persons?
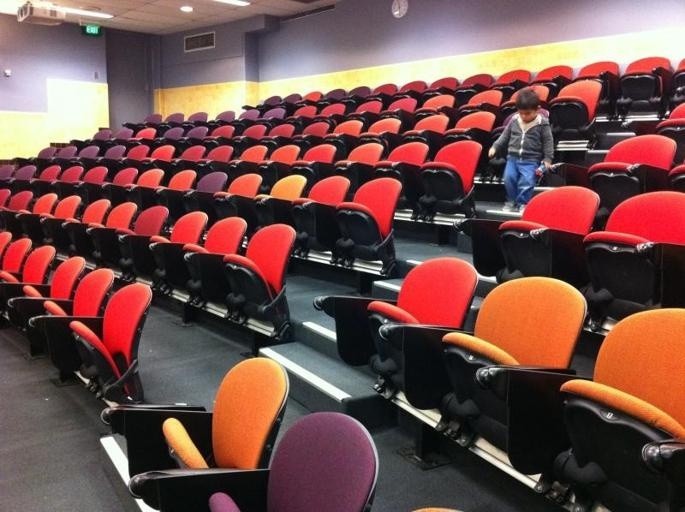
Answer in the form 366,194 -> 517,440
486,89 -> 553,214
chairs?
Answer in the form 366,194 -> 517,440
0,55 -> 685,512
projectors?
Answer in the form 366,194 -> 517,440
17,1 -> 66,26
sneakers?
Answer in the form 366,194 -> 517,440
503,203 -> 519,212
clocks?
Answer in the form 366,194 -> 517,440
389,0 -> 410,19
